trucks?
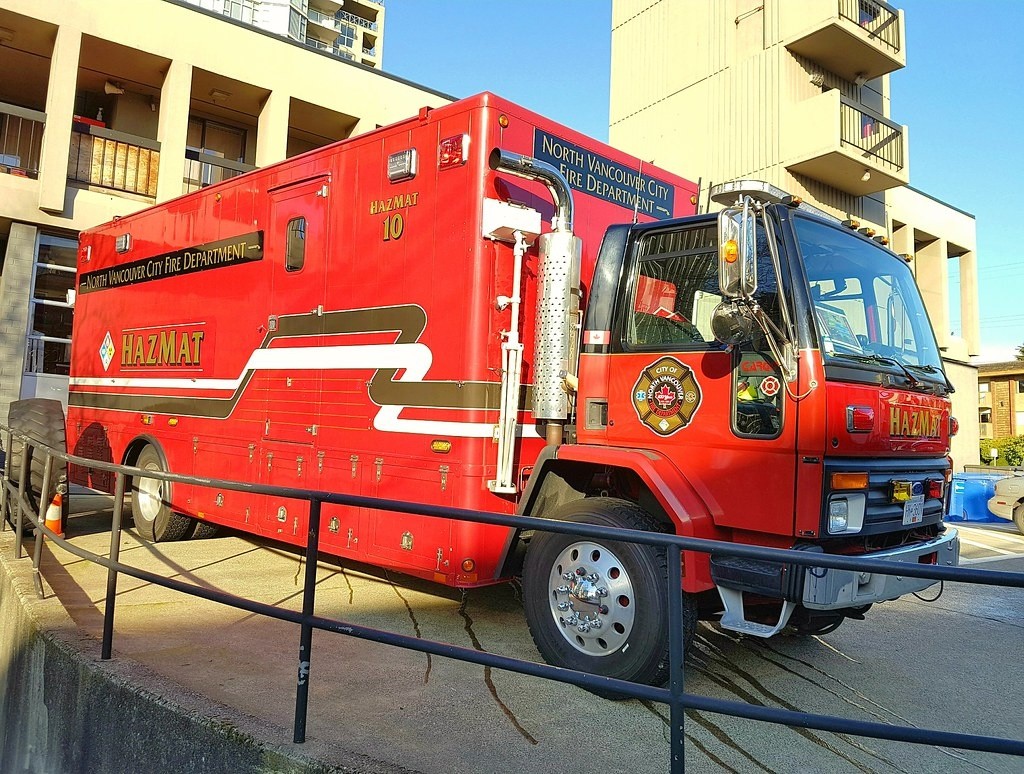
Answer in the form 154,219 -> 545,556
59,88 -> 965,703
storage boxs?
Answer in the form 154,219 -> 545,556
0,154 -> 20,168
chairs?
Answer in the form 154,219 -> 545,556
635,276 -> 705,344
